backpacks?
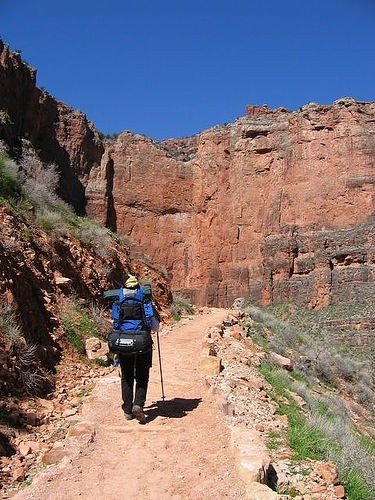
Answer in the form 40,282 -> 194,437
108,285 -> 153,353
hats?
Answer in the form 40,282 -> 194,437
125,276 -> 138,287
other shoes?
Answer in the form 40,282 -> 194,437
124,412 -> 133,420
131,405 -> 147,425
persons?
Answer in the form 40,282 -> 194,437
112,276 -> 159,424
